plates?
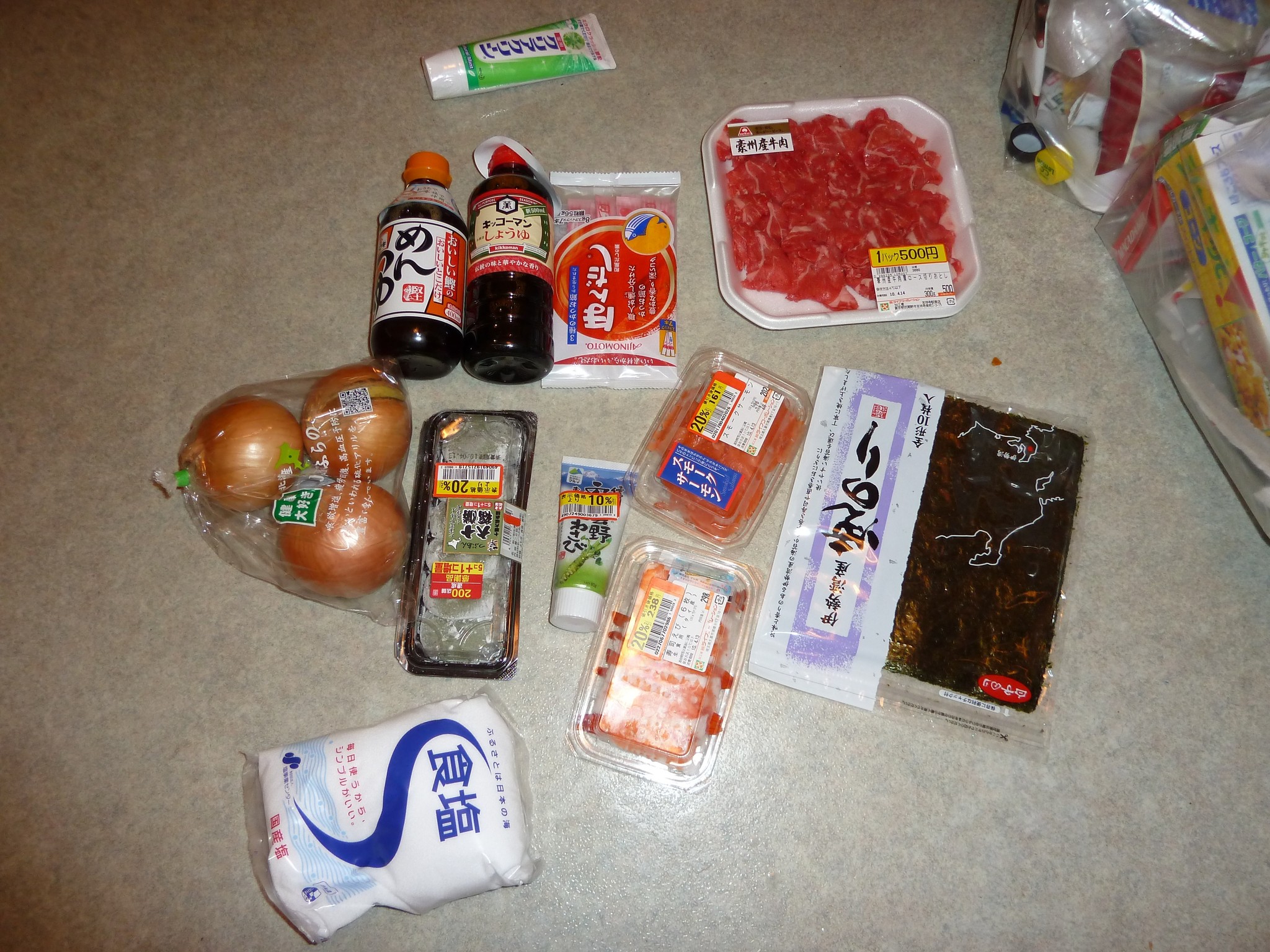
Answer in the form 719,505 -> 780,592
701,95 -> 983,331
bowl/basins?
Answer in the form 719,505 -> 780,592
622,343 -> 814,560
562,534 -> 765,792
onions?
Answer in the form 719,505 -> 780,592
177,396 -> 303,512
275,479 -> 410,596
299,364 -> 412,484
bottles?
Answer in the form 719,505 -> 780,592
461,143 -> 555,386
368,150 -> 467,380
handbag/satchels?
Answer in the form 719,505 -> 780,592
998,1 -> 1270,218
1093,86 -> 1270,546
